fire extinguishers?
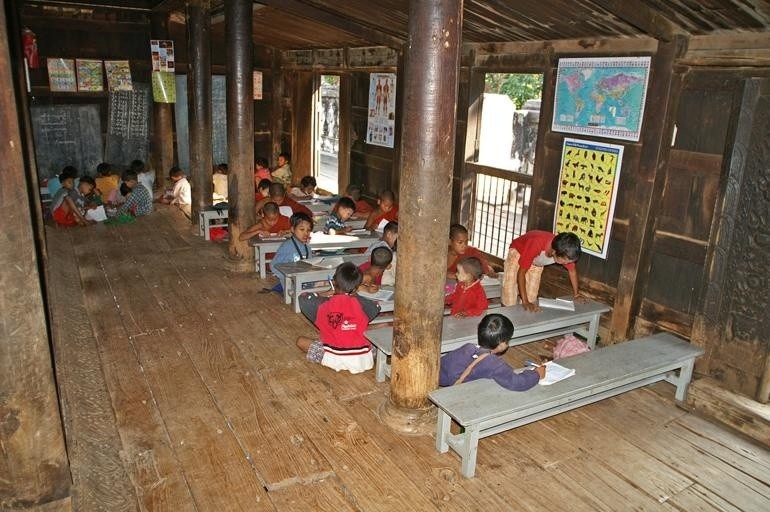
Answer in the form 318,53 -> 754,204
21,24 -> 40,69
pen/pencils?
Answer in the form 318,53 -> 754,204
526,360 -> 541,368
329,280 -> 335,290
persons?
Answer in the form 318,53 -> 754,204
357,221 -> 495,319
500,230 -> 591,313
162,166 -> 191,205
322,184 -> 374,234
363,191 -> 399,231
239,154 -> 317,272
269,211 -> 331,297
213,163 -> 228,199
47,158 -> 155,229
297,262 -> 382,375
440,313 -> 547,392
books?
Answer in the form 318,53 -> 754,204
525,359 -> 577,387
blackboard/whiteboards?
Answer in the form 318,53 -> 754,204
175,75 -> 228,175
104,82 -> 152,167
30,104 -> 103,183
